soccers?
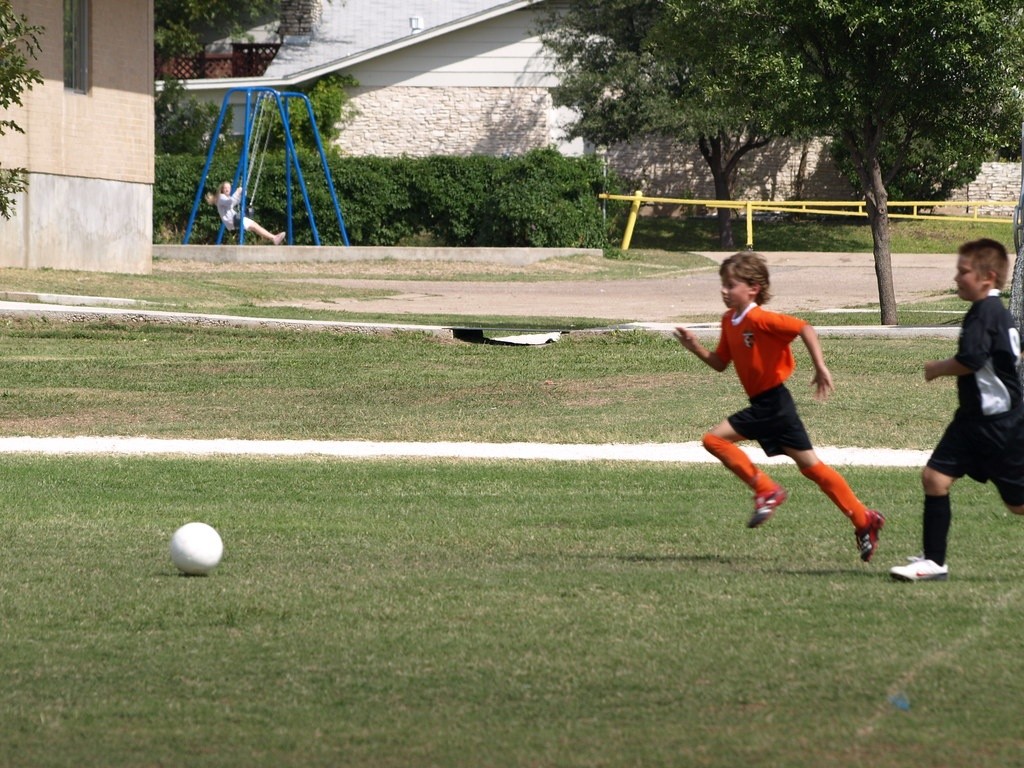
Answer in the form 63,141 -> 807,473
167,521 -> 224,575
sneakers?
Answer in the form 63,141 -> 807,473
889,552 -> 948,581
854,510 -> 885,562
746,485 -> 785,528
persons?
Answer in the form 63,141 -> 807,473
671,251 -> 885,562
888,237 -> 1024,583
213,181 -> 286,245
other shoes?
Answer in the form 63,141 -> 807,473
274,232 -> 286,245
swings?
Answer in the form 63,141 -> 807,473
228,96 -> 277,233
